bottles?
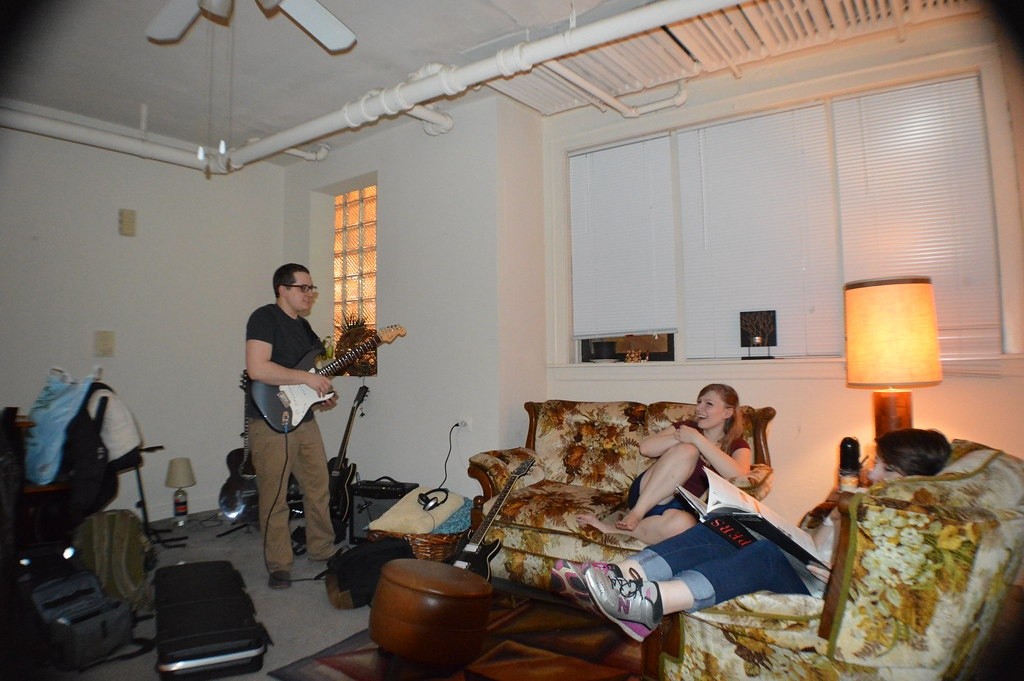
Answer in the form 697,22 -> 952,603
837,469 -> 858,493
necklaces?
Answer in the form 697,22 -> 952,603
700,455 -> 710,466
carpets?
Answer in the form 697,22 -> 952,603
265,572 -> 682,680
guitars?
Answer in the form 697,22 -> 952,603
442,460 -> 534,579
219,369 -> 260,525
250,324 -> 407,433
328,386 -> 368,519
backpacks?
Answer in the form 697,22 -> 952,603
314,534 -> 417,610
18,548 -> 154,675
74,510 -> 151,604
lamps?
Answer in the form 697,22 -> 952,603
164,455 -> 198,522
840,274 -> 940,437
199,0 -> 286,20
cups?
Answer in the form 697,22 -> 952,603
592,341 -> 617,360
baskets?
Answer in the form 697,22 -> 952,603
363,528 -> 470,562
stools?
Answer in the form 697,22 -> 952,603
365,557 -> 495,667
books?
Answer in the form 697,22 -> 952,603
675,466 -> 833,582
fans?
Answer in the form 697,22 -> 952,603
143,0 -> 360,55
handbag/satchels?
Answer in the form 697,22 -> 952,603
23,366 -> 95,488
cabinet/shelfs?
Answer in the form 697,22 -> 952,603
15,412 -> 62,494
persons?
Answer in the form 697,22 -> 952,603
245,263 -> 340,588
551,428 -> 953,641
574,383 -> 751,545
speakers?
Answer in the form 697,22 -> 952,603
349,475 -> 419,546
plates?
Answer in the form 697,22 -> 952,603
589,359 -> 620,362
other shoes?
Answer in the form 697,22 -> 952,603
267,569 -> 292,590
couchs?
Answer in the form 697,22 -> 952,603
659,436 -> 1024,681
468,398 -> 777,609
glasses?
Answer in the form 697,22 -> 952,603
281,284 -> 318,293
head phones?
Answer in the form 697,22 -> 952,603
418,488 -> 448,511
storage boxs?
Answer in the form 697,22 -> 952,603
23,567 -> 135,668
152,560 -> 276,681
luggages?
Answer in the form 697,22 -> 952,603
155,561 -> 276,681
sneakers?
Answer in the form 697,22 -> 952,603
551,559 -> 623,616
585,567 -> 664,643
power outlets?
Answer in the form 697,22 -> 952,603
92,331 -> 117,359
119,208 -> 138,236
456,416 -> 473,432
